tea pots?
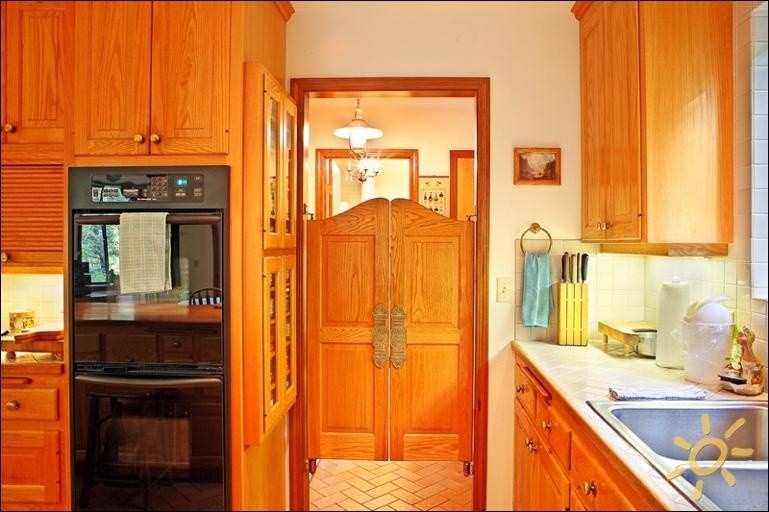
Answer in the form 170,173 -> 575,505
683,295 -> 736,386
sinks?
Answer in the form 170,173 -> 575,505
651,461 -> 769,512
586,397 -> 768,461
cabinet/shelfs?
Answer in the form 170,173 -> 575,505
0,1 -> 295,511
511,2 -> 769,512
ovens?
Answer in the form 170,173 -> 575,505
68,166 -> 229,512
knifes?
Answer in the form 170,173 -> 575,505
561,251 -> 589,283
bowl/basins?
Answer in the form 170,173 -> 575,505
632,331 -> 657,357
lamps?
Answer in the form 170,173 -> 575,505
333,97 -> 383,185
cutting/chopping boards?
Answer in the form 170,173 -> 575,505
14,321 -> 64,344
